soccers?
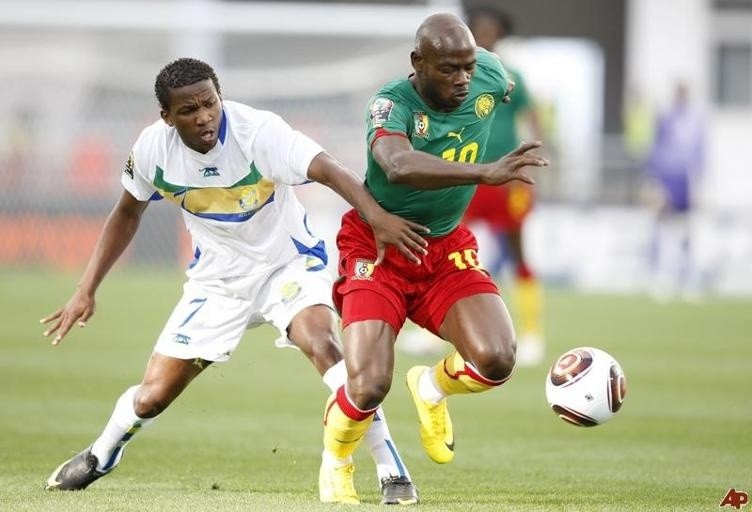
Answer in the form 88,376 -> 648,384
545,346 -> 626,427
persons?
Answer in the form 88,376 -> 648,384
633,71 -> 710,298
39,57 -> 432,506
392,6 -> 551,369
314,12 -> 551,507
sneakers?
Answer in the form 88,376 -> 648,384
380,475 -> 419,506
405,365 -> 454,463
45,444 -> 124,491
318,458 -> 358,503
